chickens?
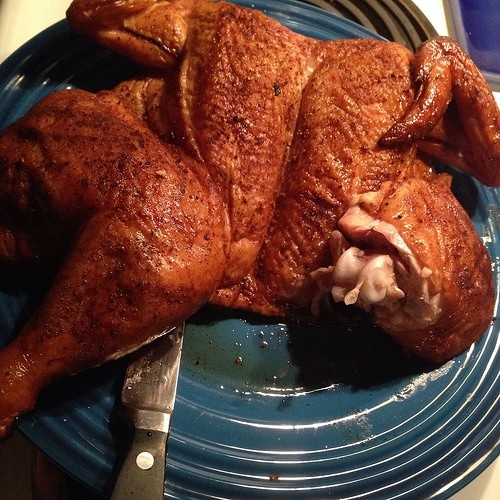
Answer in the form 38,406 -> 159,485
0,0 -> 500,436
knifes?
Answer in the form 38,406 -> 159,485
110,321 -> 185,500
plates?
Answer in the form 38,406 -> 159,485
1,1 -> 499,499
